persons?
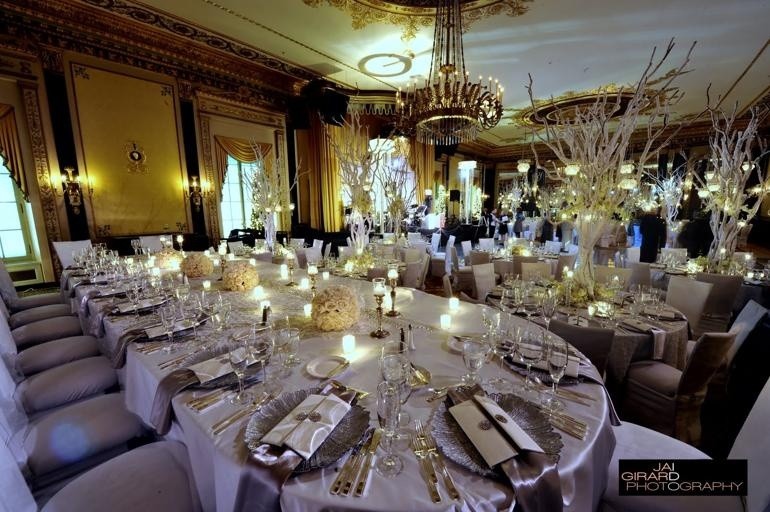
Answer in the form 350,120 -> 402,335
414,212 -> 421,226
445,217 -> 449,228
540,200 -> 579,243
449,215 -> 459,228
480,206 -> 525,238
679,209 -> 715,257
638,205 -> 668,263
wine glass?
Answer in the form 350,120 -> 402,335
67,234 -> 597,506
652,252 -> 769,285
599,270 -> 676,337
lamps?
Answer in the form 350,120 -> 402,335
51,167 -> 94,215
393,1 -> 504,147
697,163 -> 756,199
517,126 -> 531,174
182,175 -> 211,213
620,148 -> 634,175
617,174 -> 637,190
